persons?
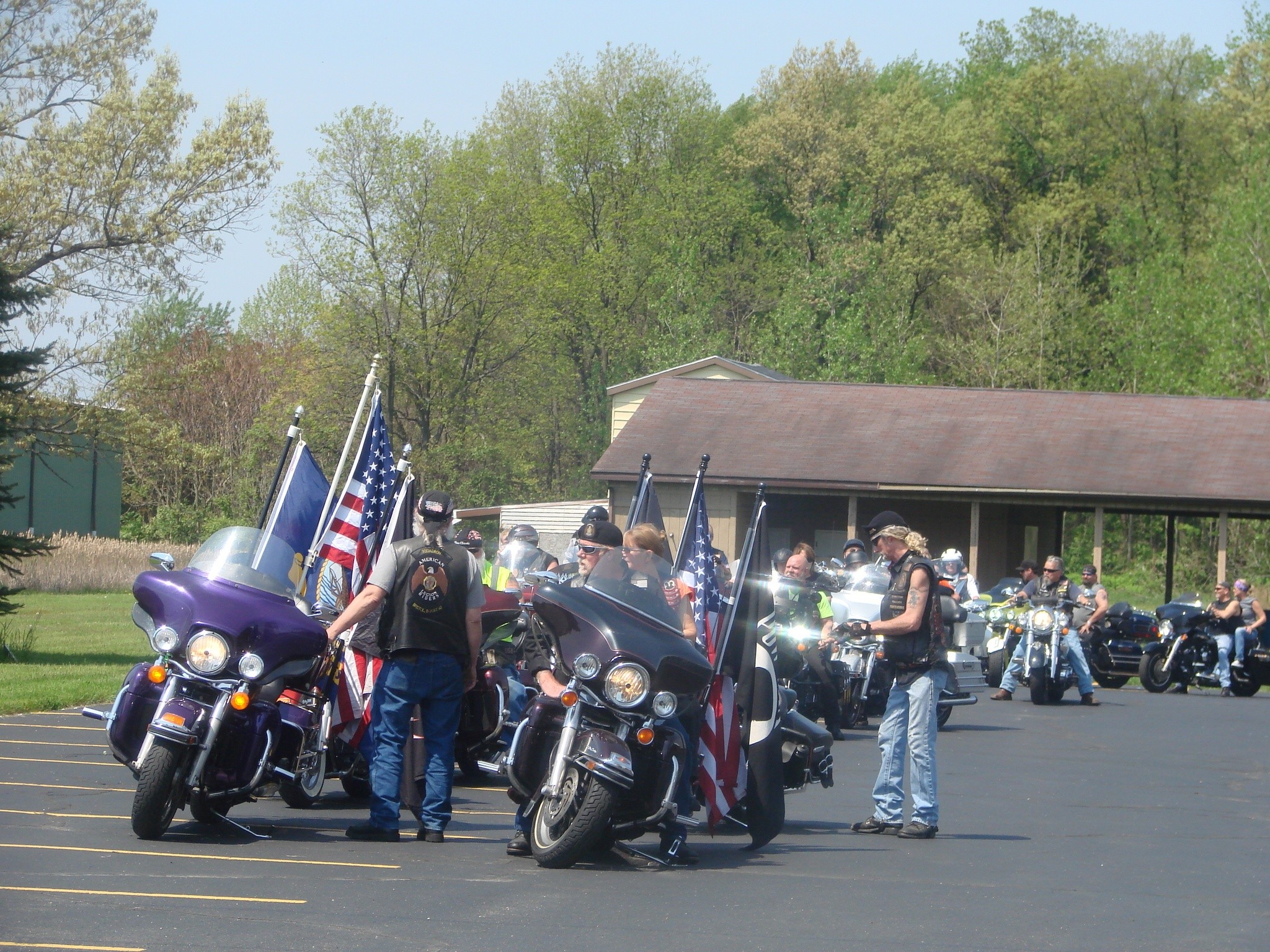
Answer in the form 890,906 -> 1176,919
990,556 -> 1100,706
826,512 -> 948,839
1166,581 -> 1243,697
1231,578 -> 1266,669
708,525 -> 732,584
1016,558 -> 1039,585
772,539 -> 982,741
326,491 -> 485,844
453,505 -> 701,865
1071,565 -> 1108,688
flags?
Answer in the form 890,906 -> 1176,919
252,381 -> 425,822
628,470 -> 786,850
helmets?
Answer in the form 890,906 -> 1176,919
581,506 -> 609,524
940,548 -> 963,567
508,524 -> 538,547
708,525 -> 714,541
845,551 -> 868,566
843,539 -> 865,553
773,548 -> 793,571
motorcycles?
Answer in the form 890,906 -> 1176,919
832,564 -> 988,730
478,546 -> 749,869
80,526 -> 362,841
959,592 -> 1270,706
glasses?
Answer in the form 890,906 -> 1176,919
850,549 -> 861,553
468,548 -> 481,553
871,534 -> 882,546
624,548 -> 643,554
807,558 -> 813,562
1214,588 -> 1225,590
1081,573 -> 1093,576
1043,568 -> 1059,573
578,544 -> 607,555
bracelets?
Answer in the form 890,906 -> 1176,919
1087,621 -> 1092,626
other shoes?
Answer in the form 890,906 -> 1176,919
989,687 -> 1013,701
1231,660 -> 1244,668
1168,686 -> 1188,694
345,821 -> 445,842
851,817 -> 937,839
1081,693 -> 1100,705
690,795 -> 701,812
1221,687 -> 1230,697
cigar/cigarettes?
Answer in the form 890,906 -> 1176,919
874,555 -> 883,566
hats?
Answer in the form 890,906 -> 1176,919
1015,559 -> 1038,571
453,528 -> 483,548
864,511 -> 908,541
417,490 -> 454,521
580,521 -> 623,547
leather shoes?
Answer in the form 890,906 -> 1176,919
660,839 -> 699,863
506,829 -> 532,855
